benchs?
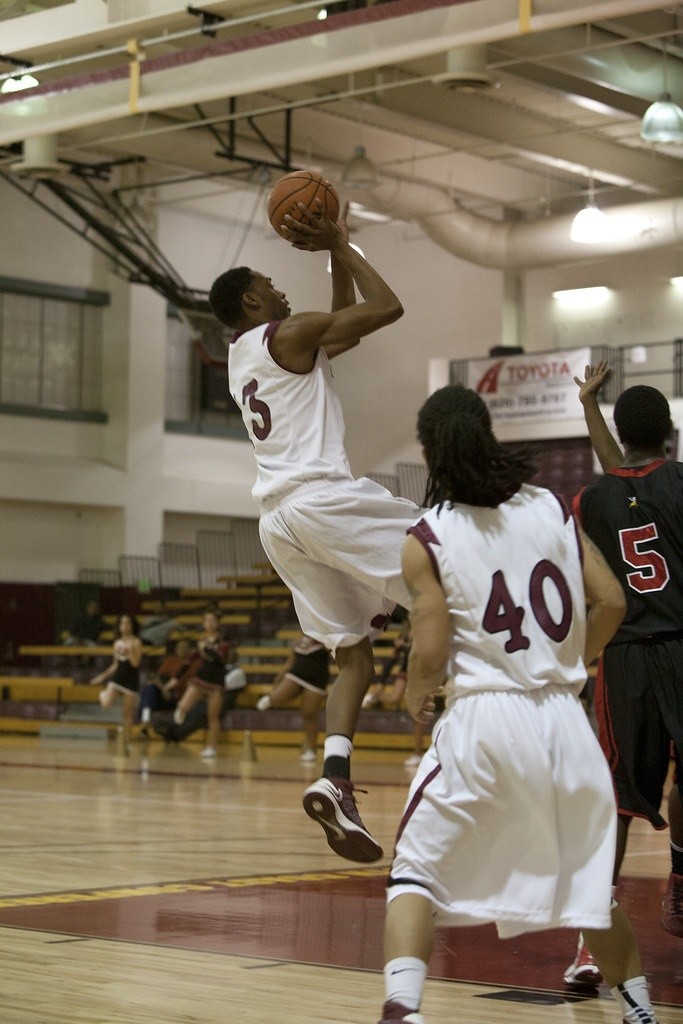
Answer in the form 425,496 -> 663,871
0,561 -> 602,752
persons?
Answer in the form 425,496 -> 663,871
380,618 -> 428,767
572,385 -> 682,939
571,359 -> 624,474
86,609 -> 330,765
205,197 -> 431,867
558,386 -> 683,990
378,385 -> 661,1024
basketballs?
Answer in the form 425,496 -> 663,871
267,170 -> 340,243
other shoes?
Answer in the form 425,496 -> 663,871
377,1002 -> 424,1024
562,943 -> 603,985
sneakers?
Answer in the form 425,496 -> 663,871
660,872 -> 683,938
302,776 -> 383,863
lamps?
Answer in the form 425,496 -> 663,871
640,38 -> 683,143
571,170 -> 606,244
